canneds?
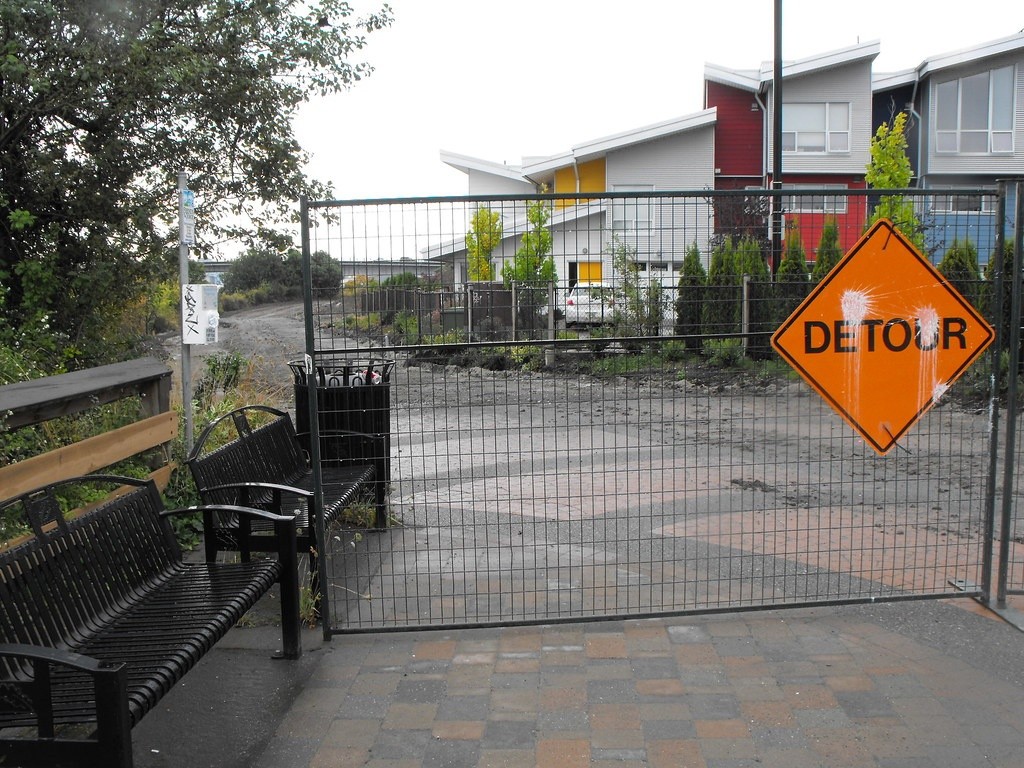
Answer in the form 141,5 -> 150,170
362,369 -> 380,384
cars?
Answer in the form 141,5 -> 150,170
564,281 -> 622,329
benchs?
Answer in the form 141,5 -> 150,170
1,475 -> 303,768
183,405 -> 389,618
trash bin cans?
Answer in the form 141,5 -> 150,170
286,357 -> 397,531
